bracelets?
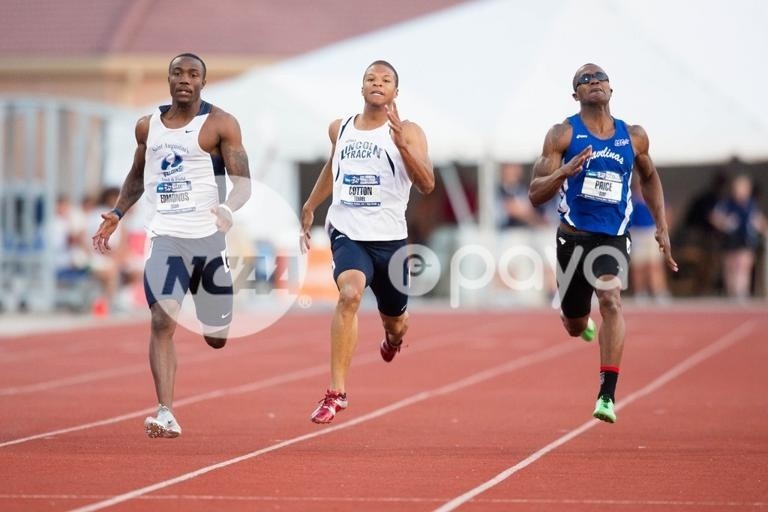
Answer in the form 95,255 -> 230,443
217,201 -> 235,217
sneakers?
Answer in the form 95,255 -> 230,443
592,395 -> 616,423
380,331 -> 403,363
143,411 -> 182,439
311,389 -> 347,424
580,317 -> 596,341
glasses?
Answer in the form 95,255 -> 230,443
575,72 -> 609,92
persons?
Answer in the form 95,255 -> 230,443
528,62 -> 681,424
299,59 -> 438,425
90,53 -> 253,439
53,183 -> 146,314
502,160 -> 767,297
